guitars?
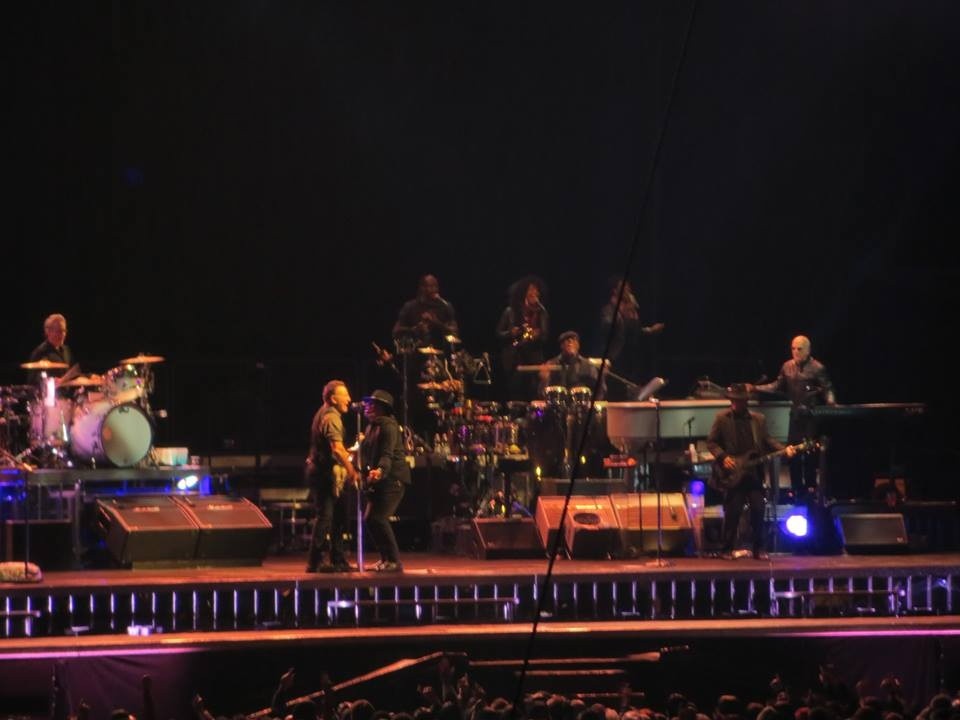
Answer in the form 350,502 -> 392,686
708,435 -> 828,498
644,318 -> 667,335
332,429 -> 365,499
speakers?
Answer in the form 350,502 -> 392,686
472,490 -> 692,560
835,513 -> 909,554
91,494 -> 273,566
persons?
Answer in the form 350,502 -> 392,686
745,333 -> 835,498
0,660 -> 960,720
537,330 -> 608,402
707,382 -> 797,561
25,314 -> 85,401
391,273 -> 459,445
303,380 -> 363,573
598,272 -> 664,402
494,274 -> 549,403
356,389 -> 411,572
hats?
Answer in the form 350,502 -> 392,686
722,382 -> 751,400
363,389 -> 394,412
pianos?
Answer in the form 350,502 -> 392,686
606,400 -> 795,499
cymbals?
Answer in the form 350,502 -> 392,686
446,336 -> 460,342
60,376 -> 103,387
23,359 -> 67,370
119,356 -> 166,365
413,345 -> 436,356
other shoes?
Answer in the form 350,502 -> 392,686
373,561 -> 403,572
720,551 -> 736,559
91,559 -> 131,570
754,551 -> 770,561
306,564 -> 335,573
334,564 -> 353,572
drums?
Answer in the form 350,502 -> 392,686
100,403 -> 152,468
113,376 -> 147,401
29,398 -> 71,448
542,385 -> 568,412
71,400 -> 118,464
570,385 -> 592,414
461,415 -> 493,465
495,418 -> 531,463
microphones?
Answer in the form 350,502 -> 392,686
683,417 -> 695,425
432,293 -> 447,306
351,401 -> 366,410
